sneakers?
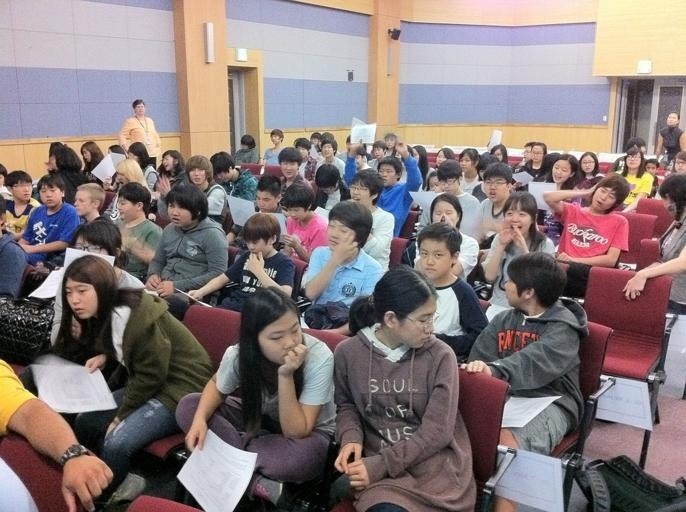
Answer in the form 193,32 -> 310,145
106,472 -> 146,504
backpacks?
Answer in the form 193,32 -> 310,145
576,455 -> 686,512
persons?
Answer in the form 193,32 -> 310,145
1,128 -> 686,510
654,112 -> 685,160
116,99 -> 162,169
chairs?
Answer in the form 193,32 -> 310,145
0,149 -> 686,512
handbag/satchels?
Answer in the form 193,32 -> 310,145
0,295 -> 54,365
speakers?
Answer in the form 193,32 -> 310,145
391,28 -> 401,40
386,46 -> 393,75
206,22 -> 214,63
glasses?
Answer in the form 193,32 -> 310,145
501,280 -> 509,288
70,242 -> 102,253
627,155 -> 642,161
484,179 -> 505,187
439,179 -> 456,185
674,161 -> 686,166
317,186 -> 333,194
405,312 -> 440,329
349,184 -> 368,193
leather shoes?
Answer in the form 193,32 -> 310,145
276,479 -> 331,512
171,449 -> 192,468
